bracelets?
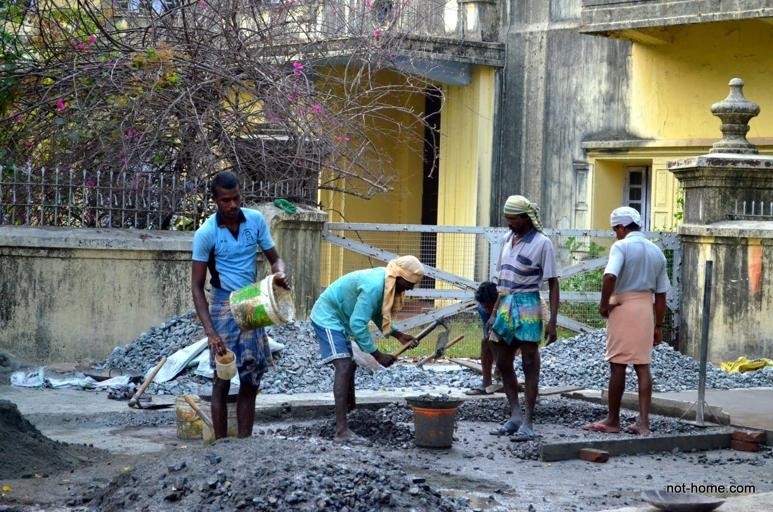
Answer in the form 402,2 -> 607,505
655,323 -> 662,328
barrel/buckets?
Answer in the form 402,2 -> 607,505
200,400 -> 239,440
414,406 -> 456,450
174,396 -> 202,439
229,274 -> 295,334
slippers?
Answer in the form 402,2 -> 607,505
489,421 -> 545,442
465,387 -> 494,395
581,418 -> 651,436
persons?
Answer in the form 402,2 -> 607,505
462,280 -> 523,395
579,205 -> 671,438
306,253 -> 426,448
188,170 -> 293,441
485,193 -> 561,443
